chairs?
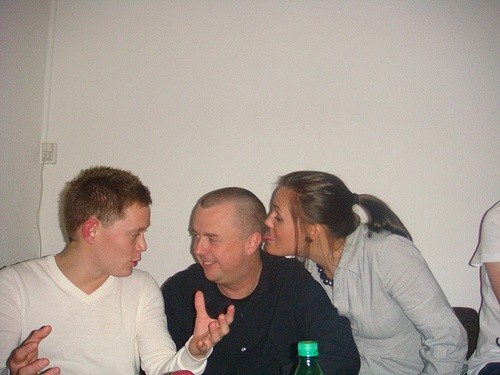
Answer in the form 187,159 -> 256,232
452,307 -> 479,362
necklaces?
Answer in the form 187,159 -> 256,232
316,264 -> 333,286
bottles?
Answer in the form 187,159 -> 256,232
294,341 -> 323,375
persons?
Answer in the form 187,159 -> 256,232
0,167 -> 235,375
140,187 -> 361,375
469,201 -> 500,375
262,171 -> 468,375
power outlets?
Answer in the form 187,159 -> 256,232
40,143 -> 57,164
